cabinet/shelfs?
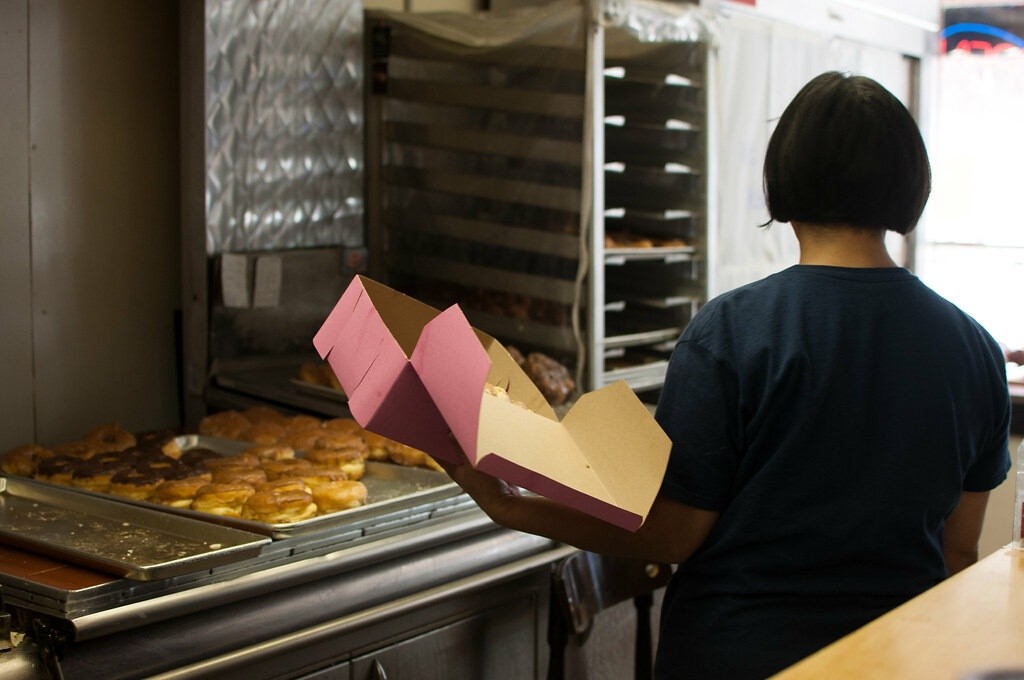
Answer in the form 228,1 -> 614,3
298,593 -> 539,680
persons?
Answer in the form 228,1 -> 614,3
429,72 -> 1013,680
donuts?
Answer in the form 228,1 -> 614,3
0,405 -> 445,528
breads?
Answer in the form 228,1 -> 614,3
605,229 -> 691,250
297,345 -> 574,406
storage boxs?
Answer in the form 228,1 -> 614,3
311,272 -> 672,533
